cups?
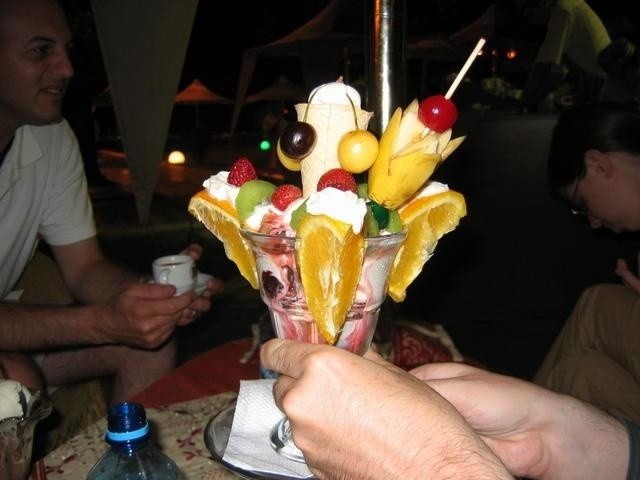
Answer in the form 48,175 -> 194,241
149,254 -> 198,299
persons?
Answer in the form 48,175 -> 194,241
2,0 -> 227,459
259,338 -> 640,479
528,89 -> 640,427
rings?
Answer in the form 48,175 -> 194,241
191,308 -> 196,319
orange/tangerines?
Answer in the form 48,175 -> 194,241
386,187 -> 468,304
187,187 -> 263,289
295,209 -> 368,347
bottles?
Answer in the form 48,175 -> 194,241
85,398 -> 185,479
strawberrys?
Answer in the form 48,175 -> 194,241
228,158 -> 257,186
316,168 -> 357,194
272,185 -> 302,211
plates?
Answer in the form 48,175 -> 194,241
144,272 -> 215,299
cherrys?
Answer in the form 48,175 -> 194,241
419,95 -> 456,133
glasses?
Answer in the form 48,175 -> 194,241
566,171 -> 587,221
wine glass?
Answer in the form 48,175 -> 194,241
237,222 -> 409,464
